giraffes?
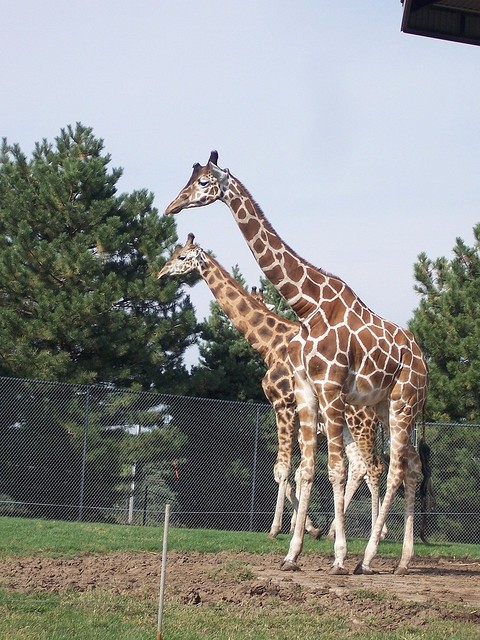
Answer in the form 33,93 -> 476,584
166,150 -> 437,575
156,232 -> 389,543
